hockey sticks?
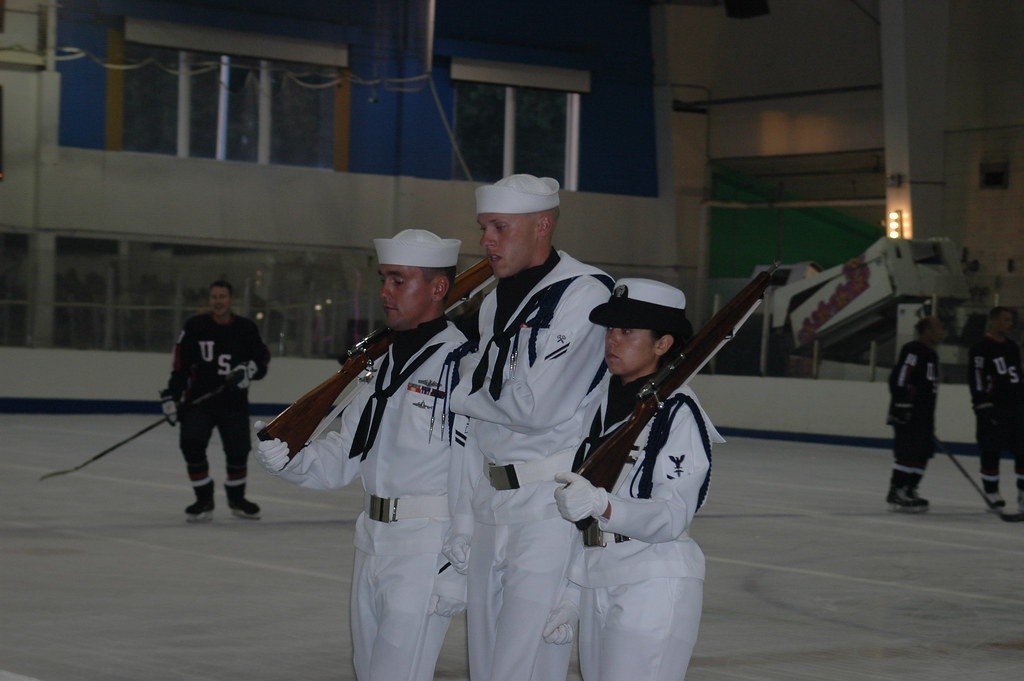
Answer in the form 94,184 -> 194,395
932,434 -> 1023,523
39,383 -> 227,481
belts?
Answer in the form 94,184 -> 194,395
580,517 -> 631,547
362,490 -> 451,523
483,450 -> 577,490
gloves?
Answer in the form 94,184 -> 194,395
229,359 -> 259,391
441,526 -> 474,575
889,397 -> 913,424
973,403 -> 995,428
255,419 -> 290,473
553,471 -> 609,523
424,571 -> 470,618
158,387 -> 181,427
542,598 -> 581,646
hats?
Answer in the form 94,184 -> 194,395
588,279 -> 687,329
376,228 -> 462,267
476,174 -> 560,213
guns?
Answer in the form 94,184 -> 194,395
565,267 -> 775,529
257,258 -> 494,458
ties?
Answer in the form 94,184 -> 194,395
468,284 -> 555,402
349,341 -> 449,462
571,405 -> 641,476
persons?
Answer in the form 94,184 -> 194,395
884,315 -> 945,508
442,173 -> 615,681
254,224 -> 470,680
159,280 -> 271,514
966,307 -> 1024,508
543,277 -> 727,681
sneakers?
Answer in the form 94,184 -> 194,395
1016,489 -> 1023,511
183,480 -> 216,523
223,476 -> 262,519
986,490 -> 1006,513
886,484 -> 932,515
